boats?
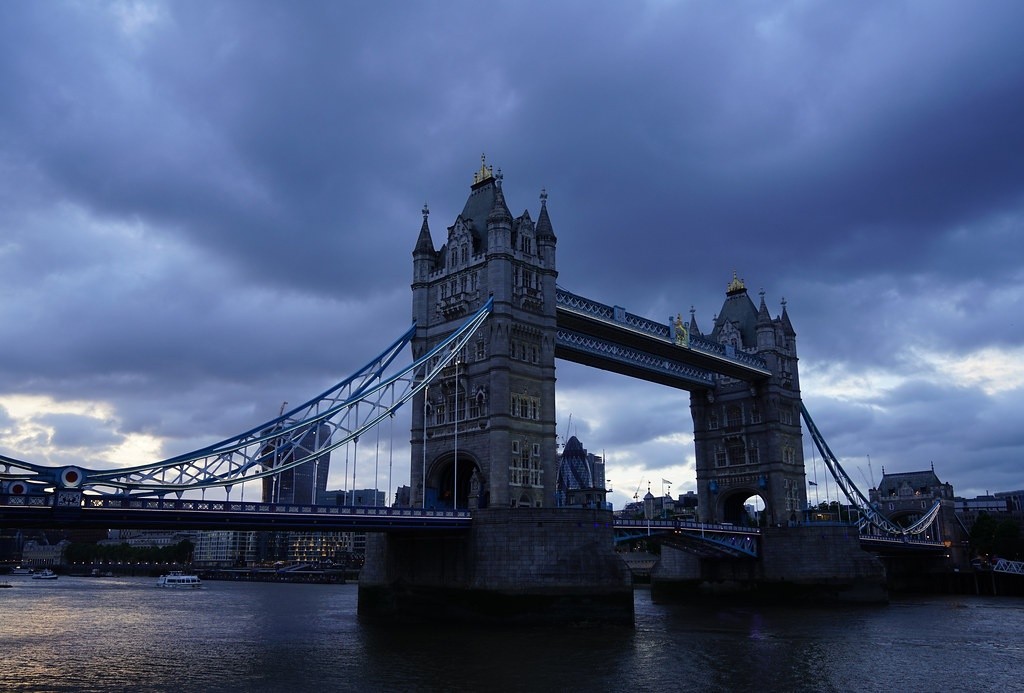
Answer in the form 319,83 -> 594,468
31,568 -> 58,579
156,570 -> 203,591
